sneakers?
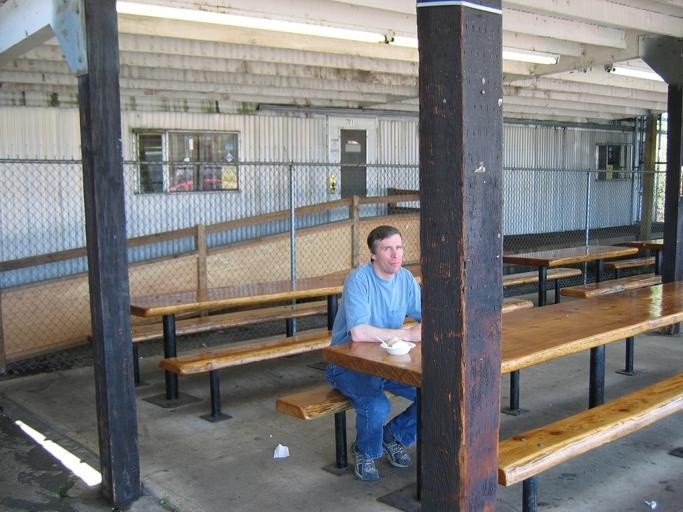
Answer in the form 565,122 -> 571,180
351,441 -> 379,481
382,440 -> 411,468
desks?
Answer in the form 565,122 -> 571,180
130,238 -> 683,512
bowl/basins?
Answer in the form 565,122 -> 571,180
380,341 -> 416,355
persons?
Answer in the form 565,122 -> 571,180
325,225 -> 422,481
